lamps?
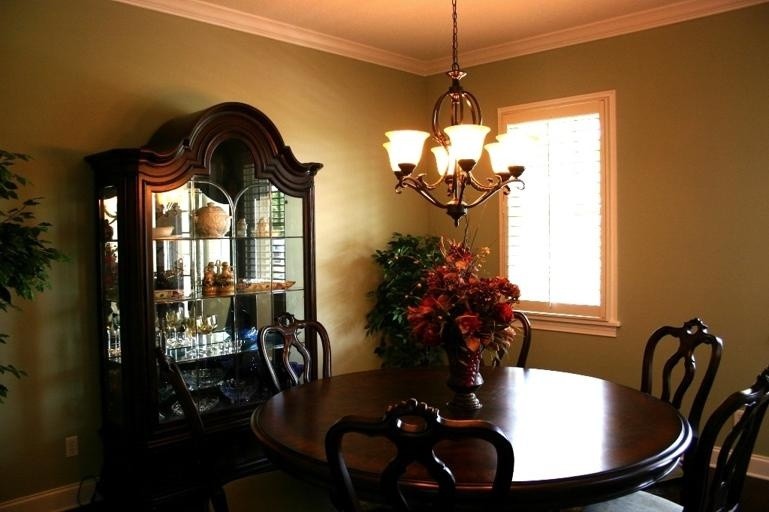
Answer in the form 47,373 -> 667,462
380,0 -> 525,226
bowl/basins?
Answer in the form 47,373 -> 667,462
156,226 -> 174,237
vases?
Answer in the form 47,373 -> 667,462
443,344 -> 485,411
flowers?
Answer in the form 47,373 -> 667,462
405,232 -> 520,357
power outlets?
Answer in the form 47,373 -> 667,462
65,436 -> 79,458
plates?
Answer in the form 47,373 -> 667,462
171,366 -> 258,416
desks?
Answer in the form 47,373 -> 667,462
248,362 -> 696,512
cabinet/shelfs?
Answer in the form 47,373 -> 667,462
82,97 -> 323,484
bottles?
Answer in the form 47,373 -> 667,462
236,218 -> 247,236
171,203 -> 181,235
104,218 -> 113,239
201,262 -> 217,297
217,262 -> 236,297
195,203 -> 232,239
256,218 -> 267,235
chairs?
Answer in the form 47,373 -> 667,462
572,362 -> 769,511
320,396 -> 514,512
489,310 -> 535,367
154,342 -> 337,511
618,315 -> 727,494
255,310 -> 335,394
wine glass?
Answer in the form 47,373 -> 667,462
165,308 -> 219,356
